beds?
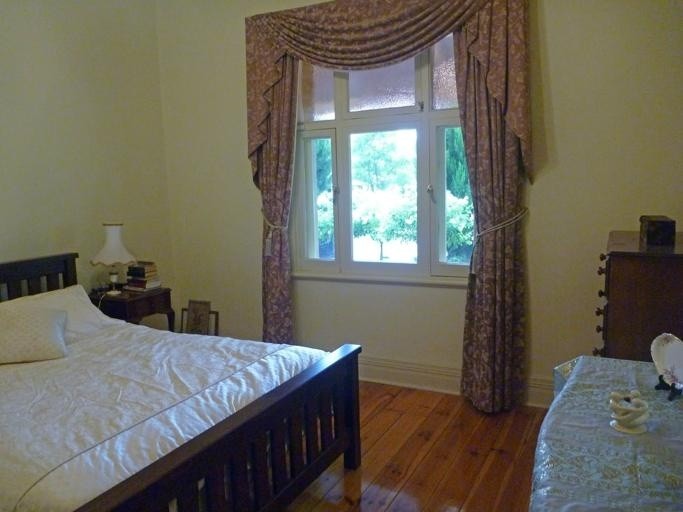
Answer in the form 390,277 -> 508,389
0,253 -> 363,512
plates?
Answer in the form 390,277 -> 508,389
650,332 -> 683,391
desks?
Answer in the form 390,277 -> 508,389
527,353 -> 683,512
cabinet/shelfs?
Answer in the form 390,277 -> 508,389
595,231 -> 683,362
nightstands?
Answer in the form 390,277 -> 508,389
89,287 -> 175,330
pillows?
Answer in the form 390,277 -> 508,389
0,282 -> 123,364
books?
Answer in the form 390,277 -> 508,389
123,261 -> 161,292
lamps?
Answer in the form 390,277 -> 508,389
89,224 -> 137,296
